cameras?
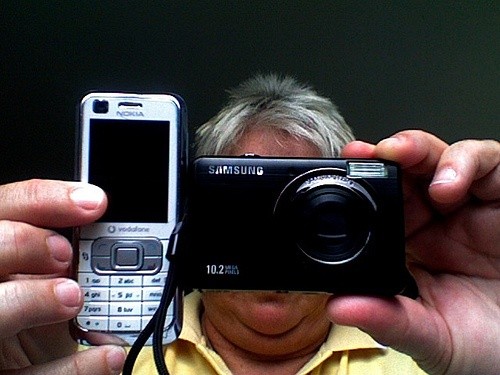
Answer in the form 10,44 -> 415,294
187,157 -> 406,296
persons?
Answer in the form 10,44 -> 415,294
0,69 -> 500,375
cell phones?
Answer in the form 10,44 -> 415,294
70,90 -> 189,349
